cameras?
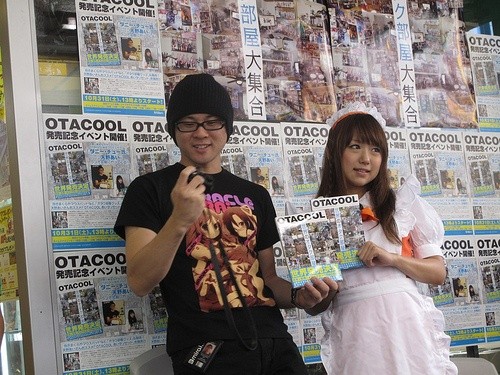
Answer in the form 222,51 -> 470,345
187,171 -> 213,194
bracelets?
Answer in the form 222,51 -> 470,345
290,288 -> 304,309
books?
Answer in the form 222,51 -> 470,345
275,194 -> 366,289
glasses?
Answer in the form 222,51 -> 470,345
174,118 -> 225,132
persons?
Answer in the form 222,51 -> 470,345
128,309 -> 142,330
144,49 -> 158,68
315,102 -> 459,375
116,175 -> 128,195
107,302 -> 120,323
124,38 -> 138,60
454,278 -> 464,297
94,165 -> 109,187
469,285 -> 480,301
114,73 -> 339,375
272,176 -> 284,191
254,169 -> 267,184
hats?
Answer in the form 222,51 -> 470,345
167,74 -> 233,136
